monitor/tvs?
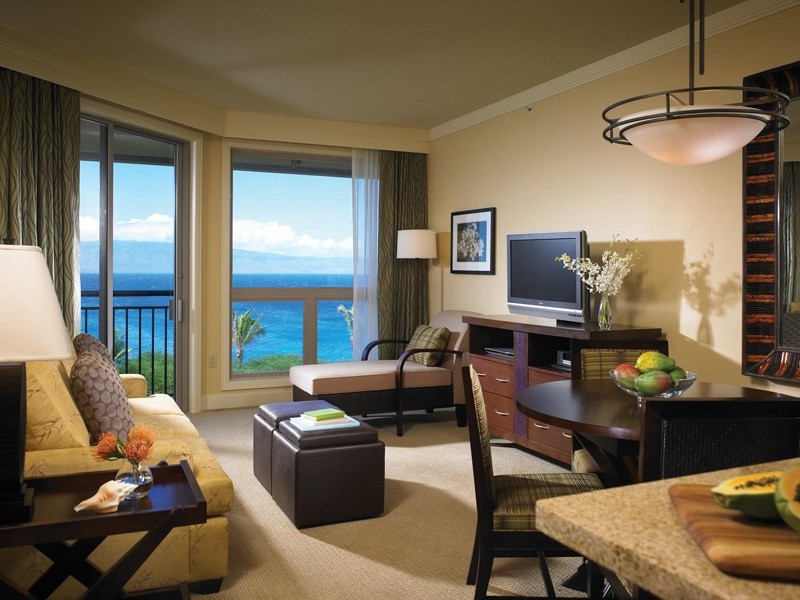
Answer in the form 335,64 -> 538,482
506,230 -> 592,324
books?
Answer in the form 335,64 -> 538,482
289,408 -> 360,433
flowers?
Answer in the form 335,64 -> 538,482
554,234 -> 643,324
89,427 -> 155,484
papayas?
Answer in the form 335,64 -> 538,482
613,352 -> 687,395
709,466 -> 800,535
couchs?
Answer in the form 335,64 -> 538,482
0,332 -> 234,595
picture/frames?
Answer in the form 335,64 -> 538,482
450,207 -> 496,275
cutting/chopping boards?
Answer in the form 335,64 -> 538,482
669,485 -> 800,575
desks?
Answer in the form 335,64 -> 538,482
535,457 -> 800,600
516,378 -> 800,593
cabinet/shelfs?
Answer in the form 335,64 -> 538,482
462,314 -> 662,473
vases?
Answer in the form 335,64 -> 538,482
114,460 -> 154,499
597,293 -> 612,329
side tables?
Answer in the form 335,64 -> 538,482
0,460 -> 206,600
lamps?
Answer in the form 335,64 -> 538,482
602,0 -> 790,166
396,229 -> 437,328
0,243 -> 77,524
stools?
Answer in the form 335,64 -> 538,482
254,399 -> 385,530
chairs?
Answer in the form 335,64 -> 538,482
404,324 -> 450,367
289,310 -> 484,436
460,340 -> 669,600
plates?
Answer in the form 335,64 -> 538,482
610,369 -> 698,408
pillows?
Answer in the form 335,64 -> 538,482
70,333 -> 135,447
